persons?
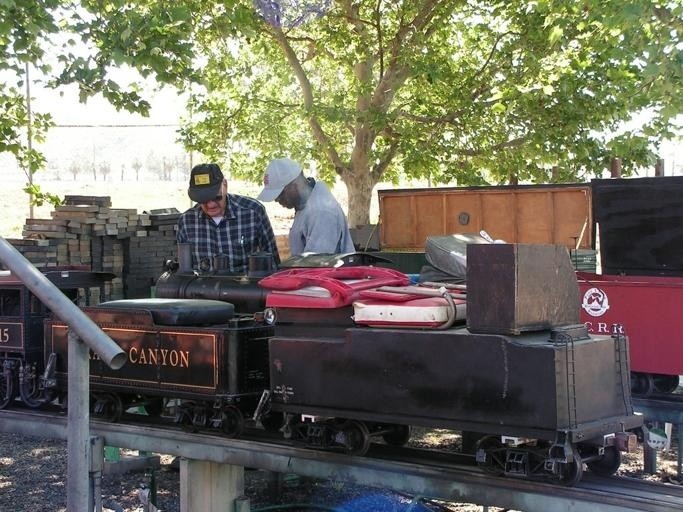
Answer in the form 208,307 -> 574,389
174,164 -> 280,272
256,158 -> 374,276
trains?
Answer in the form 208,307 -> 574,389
375,174 -> 681,400
0,240 -> 643,488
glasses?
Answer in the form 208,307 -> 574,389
198,193 -> 224,204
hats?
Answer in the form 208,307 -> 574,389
188,164 -> 223,203
257,158 -> 302,201
